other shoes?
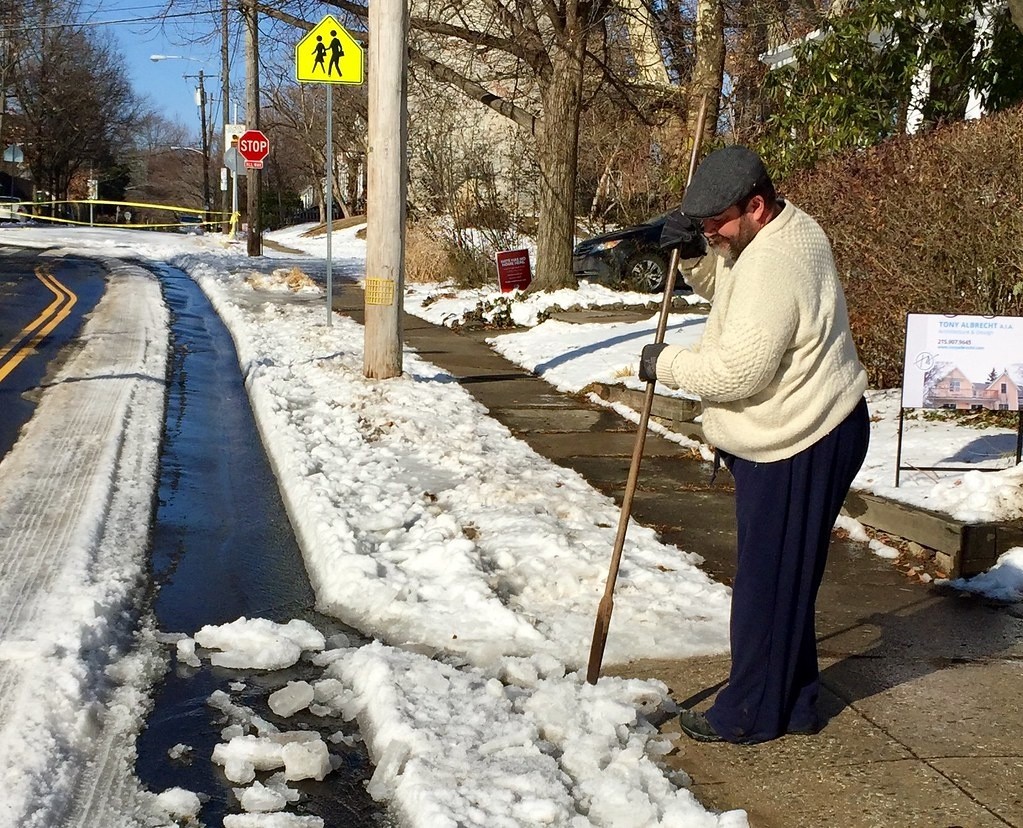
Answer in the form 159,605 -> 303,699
678,710 -> 728,743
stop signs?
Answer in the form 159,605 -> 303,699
238,130 -> 269,162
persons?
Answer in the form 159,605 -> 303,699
636,146 -> 872,746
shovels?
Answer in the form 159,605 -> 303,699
587,90 -> 710,688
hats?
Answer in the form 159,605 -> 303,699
680,144 -> 767,218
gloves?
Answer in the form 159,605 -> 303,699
639,343 -> 668,384
660,211 -> 707,259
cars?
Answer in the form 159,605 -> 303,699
0,196 -> 27,222
573,204 -> 706,293
178,215 -> 202,233
